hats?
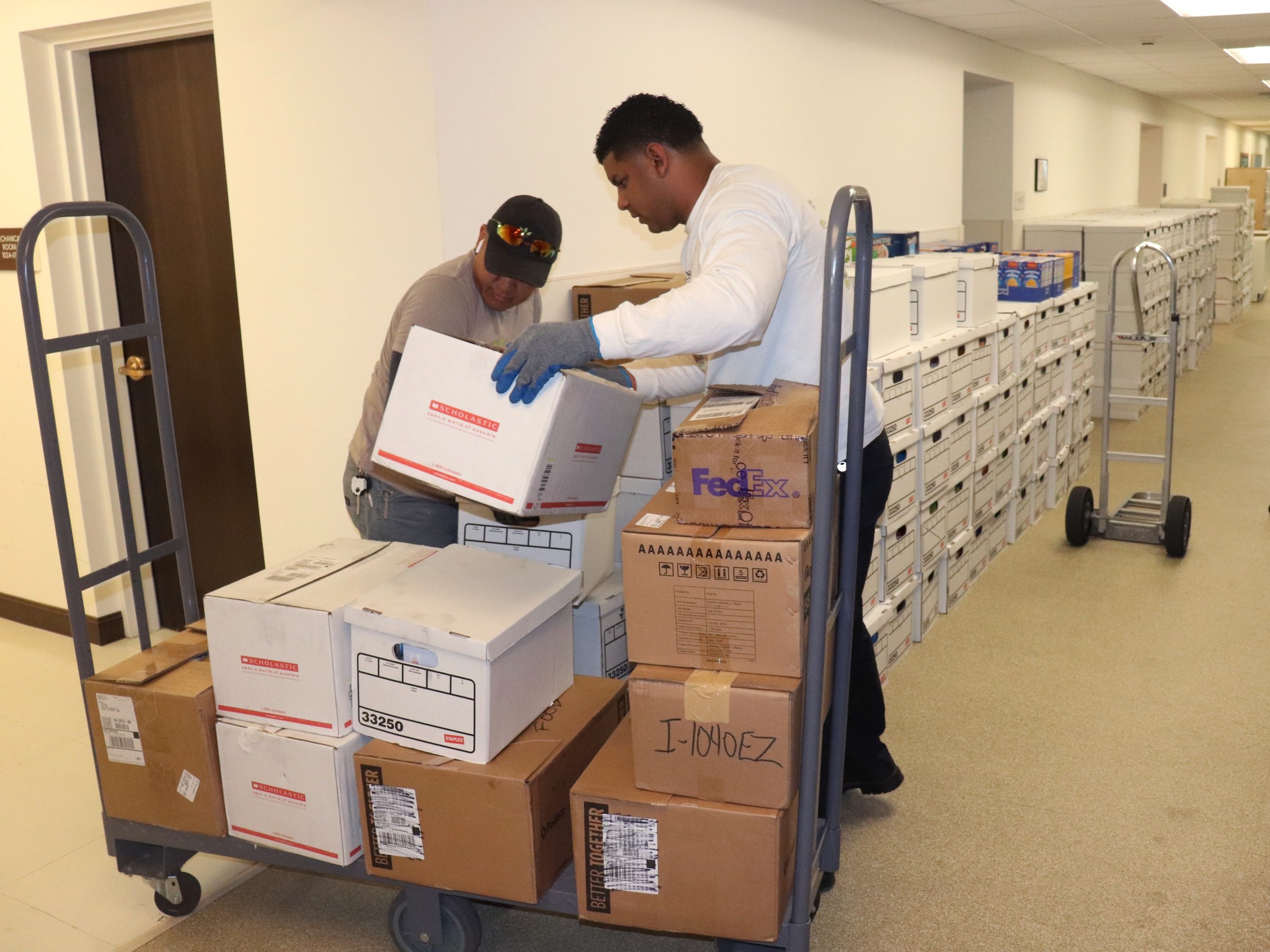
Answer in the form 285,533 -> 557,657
481,191 -> 564,292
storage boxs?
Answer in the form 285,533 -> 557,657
80,168 -> 1270,943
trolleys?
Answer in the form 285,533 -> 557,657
1064,241 -> 1192,560
17,183 -> 872,950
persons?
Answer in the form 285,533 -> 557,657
341,193 -> 566,549
487,91 -> 909,807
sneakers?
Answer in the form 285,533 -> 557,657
815,751 -> 909,803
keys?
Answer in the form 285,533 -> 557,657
351,466 -> 375,515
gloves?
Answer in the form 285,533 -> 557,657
579,358 -> 633,392
489,316 -> 604,404
